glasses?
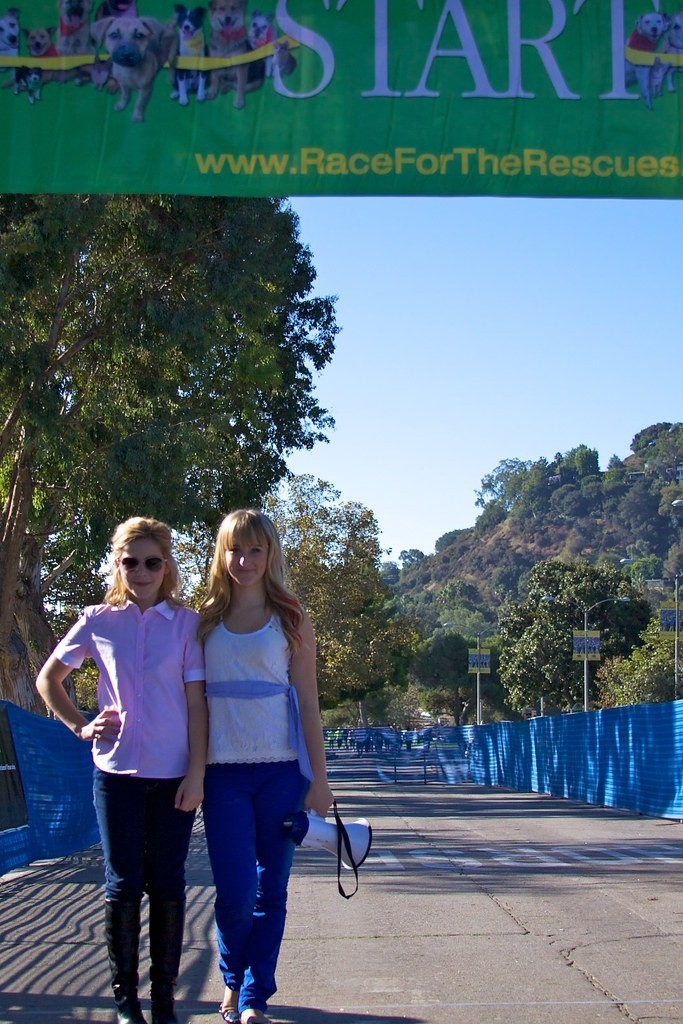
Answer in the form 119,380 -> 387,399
117,557 -> 167,573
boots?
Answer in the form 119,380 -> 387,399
104,897 -> 149,1024
146,895 -> 186,1024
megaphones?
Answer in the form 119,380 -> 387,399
283,808 -> 373,870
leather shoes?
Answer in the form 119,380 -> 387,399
239,1016 -> 271,1024
220,1003 -> 238,1024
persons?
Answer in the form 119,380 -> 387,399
32,515 -> 209,1024
193,509 -> 336,1024
323,720 -> 436,755
